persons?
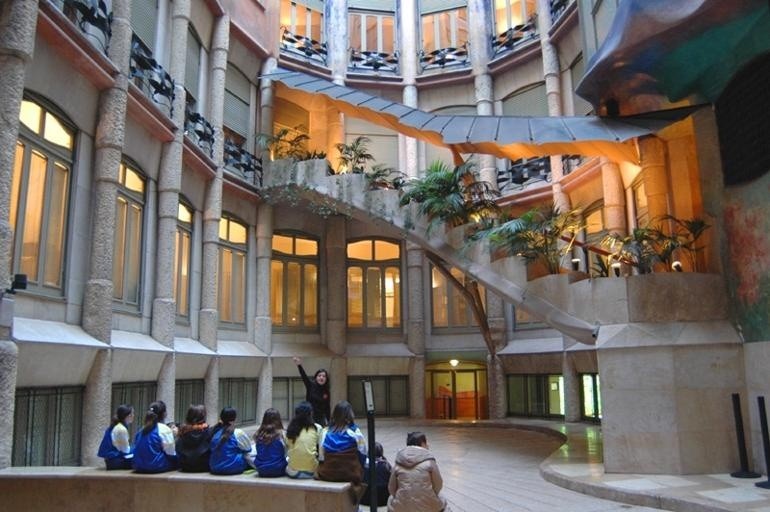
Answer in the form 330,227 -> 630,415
131,400 -> 180,473
207,407 -> 257,474
362,441 -> 393,507
387,431 -> 446,512
293,357 -> 330,428
253,408 -> 288,476
284,399 -> 323,478
318,400 -> 368,483
176,404 -> 215,472
96,404 -> 135,470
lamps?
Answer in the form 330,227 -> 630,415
610,262 -> 624,279
671,261 -> 684,271
4,273 -> 29,295
571,258 -> 581,271
